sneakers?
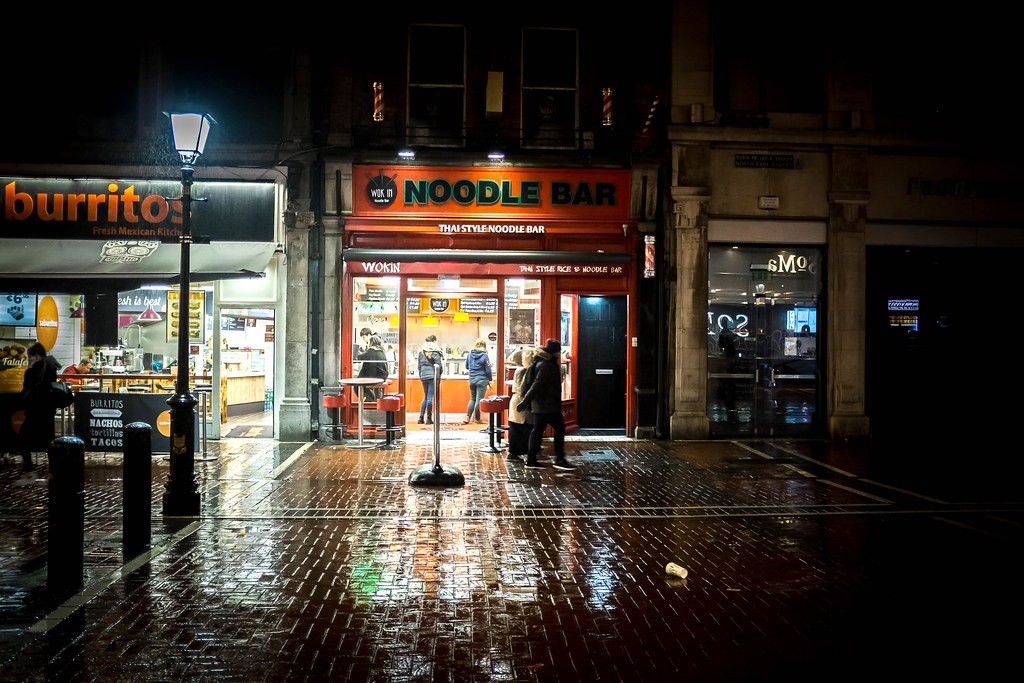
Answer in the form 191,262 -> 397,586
553,460 -> 577,471
523,460 -> 548,469
507,454 -> 521,462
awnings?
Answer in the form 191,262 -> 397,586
0,237 -> 281,277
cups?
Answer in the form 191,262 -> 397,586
665,562 -> 688,579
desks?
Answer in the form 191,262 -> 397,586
55,373 -> 212,380
338,377 -> 384,447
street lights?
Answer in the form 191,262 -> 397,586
159,102 -> 217,517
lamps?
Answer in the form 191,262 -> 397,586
421,309 -> 439,327
69,302 -> 84,319
453,309 -> 469,322
138,302 -> 163,321
390,309 -> 399,327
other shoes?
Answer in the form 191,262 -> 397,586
463,416 -> 470,423
418,416 -> 424,424
426,417 -> 432,423
475,420 -> 485,424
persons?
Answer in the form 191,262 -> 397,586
63,357 -> 91,384
463,338 -> 492,423
507,339 -> 578,470
18,342 -> 62,472
354,328 -> 415,402
718,319 -> 738,352
418,335 -> 443,424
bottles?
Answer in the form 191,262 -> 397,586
797,340 -> 802,356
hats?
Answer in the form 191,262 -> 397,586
541,338 -> 561,353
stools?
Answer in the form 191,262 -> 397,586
381,393 -> 405,444
376,396 -> 401,450
156,383 -> 176,460
194,384 -> 218,460
82,383 -> 103,391
479,398 -> 505,452
323,394 -> 348,444
365,378 -> 392,401
126,383 -> 151,392
488,394 -> 511,447
